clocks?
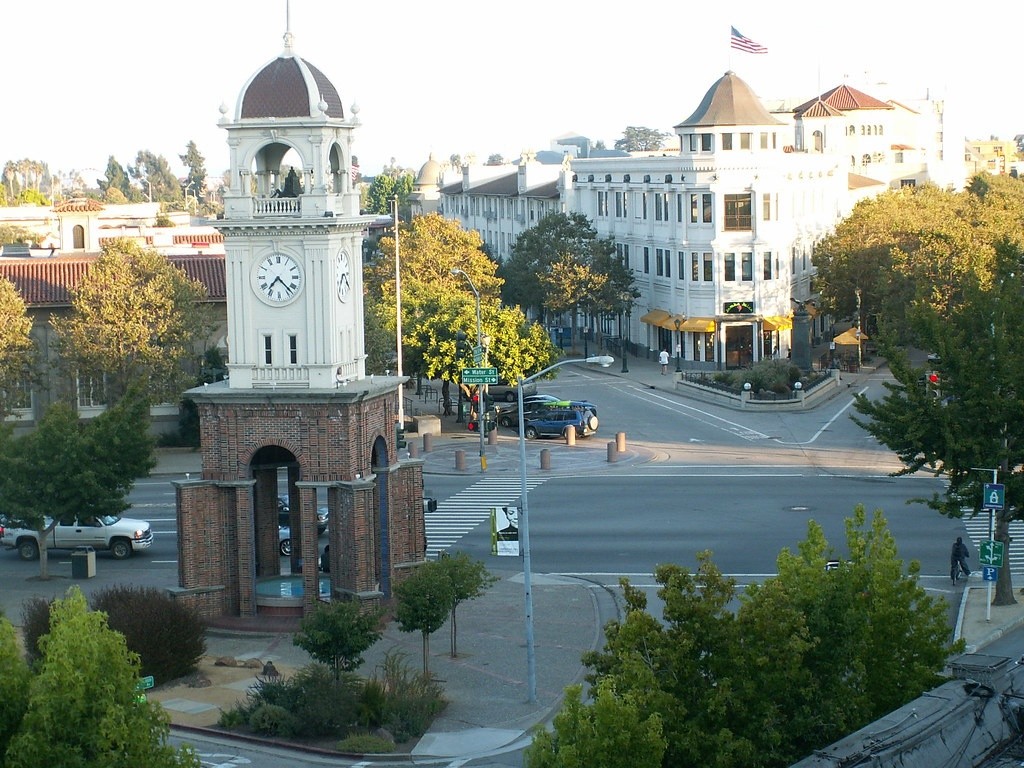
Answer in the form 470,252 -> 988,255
336,249 -> 352,302
256,252 -> 301,302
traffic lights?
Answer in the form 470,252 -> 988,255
926,370 -> 943,384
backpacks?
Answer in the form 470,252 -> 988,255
952,544 -> 962,557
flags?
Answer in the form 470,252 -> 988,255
730,26 -> 768,54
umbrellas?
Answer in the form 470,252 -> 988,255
832,326 -> 869,358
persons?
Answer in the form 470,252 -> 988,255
497,507 -> 518,541
658,348 -> 669,376
470,391 -> 479,421
772,346 -> 778,359
321,545 -> 330,573
787,349 -> 791,360
951,537 -> 971,580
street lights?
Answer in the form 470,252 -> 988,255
974,470 -> 999,623
184,181 -> 198,210
140,176 -> 154,202
450,269 -> 487,474
516,356 -> 614,707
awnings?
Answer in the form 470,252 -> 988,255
679,317 -> 715,332
763,316 -> 793,331
639,309 -> 669,327
662,314 -> 683,331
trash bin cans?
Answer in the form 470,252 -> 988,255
71,546 -> 96,579
849,356 -> 859,373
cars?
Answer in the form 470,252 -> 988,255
278,496 -> 328,558
486,376 -> 600,440
0,508 -> 154,561
